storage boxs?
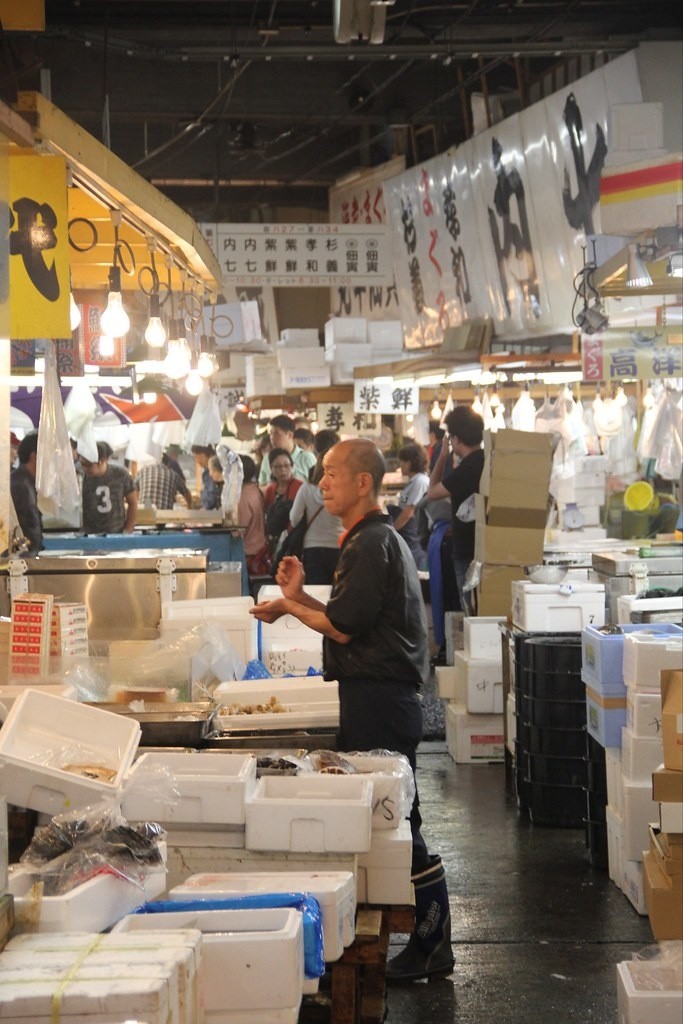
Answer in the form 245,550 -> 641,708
0,298 -> 683,1024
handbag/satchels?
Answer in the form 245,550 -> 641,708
275,529 -> 288,560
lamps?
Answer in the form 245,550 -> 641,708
668,255 -> 683,278
625,238 -> 660,290
570,239 -> 608,337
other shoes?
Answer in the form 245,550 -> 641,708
430,653 -> 447,668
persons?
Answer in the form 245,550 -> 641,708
248,439 -> 456,984
11,406 -> 485,674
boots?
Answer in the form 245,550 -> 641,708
386,854 -> 456,982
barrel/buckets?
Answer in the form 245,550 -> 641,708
623,481 -> 660,510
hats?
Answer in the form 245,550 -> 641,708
313,429 -> 340,453
